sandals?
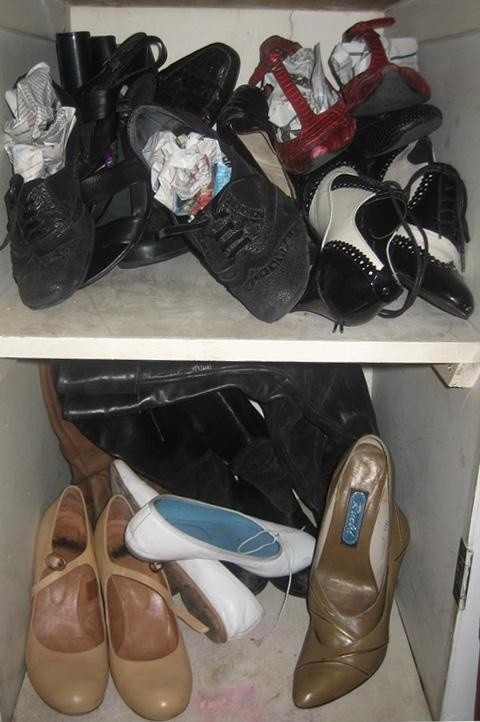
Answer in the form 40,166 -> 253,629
76,74 -> 170,294
71,32 -> 165,180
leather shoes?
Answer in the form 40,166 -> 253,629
125,492 -> 316,646
109,460 -> 265,645
25,484 -> 107,705
330,15 -> 432,117
118,205 -> 192,269
248,36 -> 356,178
306,434 -> 393,649
218,84 -> 298,205
0,64 -> 92,310
366,137 -> 475,320
160,41 -> 241,130
126,105 -> 308,324
352,106 -> 442,160
303,149 -> 429,329
291,499 -> 411,709
90,492 -> 195,717
